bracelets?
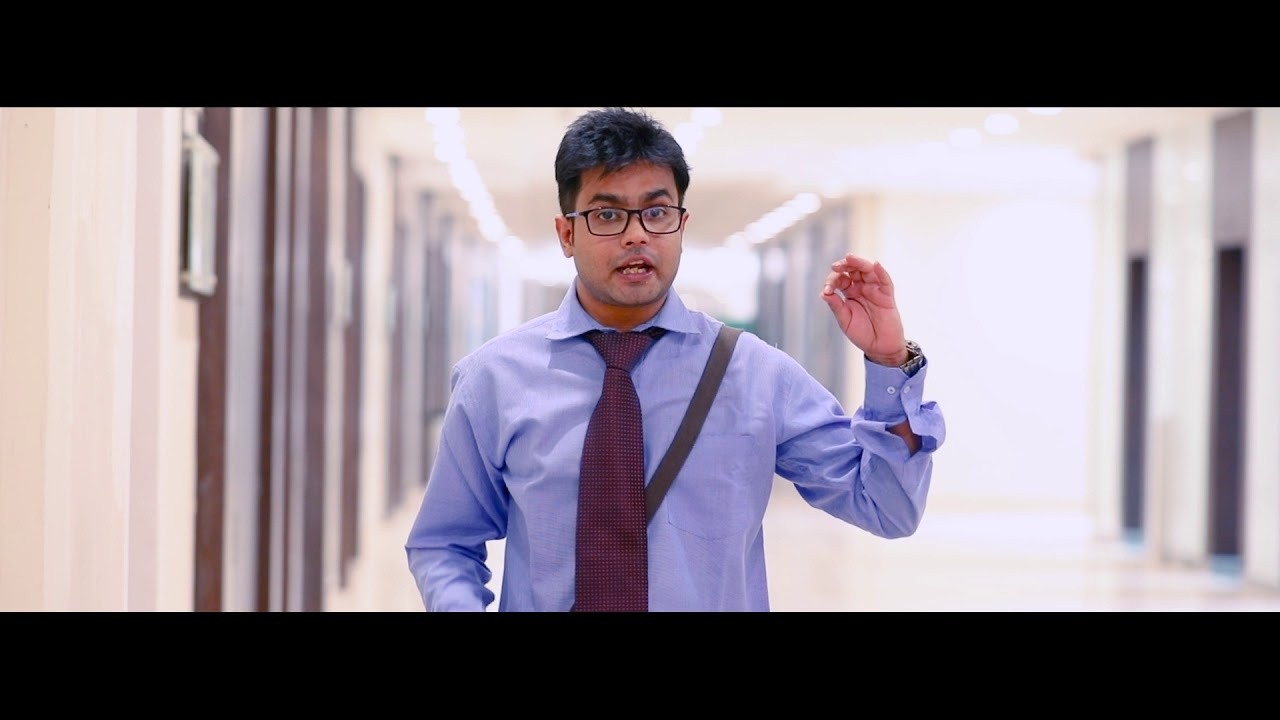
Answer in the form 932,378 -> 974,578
899,341 -> 925,377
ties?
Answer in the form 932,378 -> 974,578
573,326 -> 668,612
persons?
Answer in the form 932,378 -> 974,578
404,107 -> 946,613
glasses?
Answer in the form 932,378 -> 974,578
565,204 -> 686,236
